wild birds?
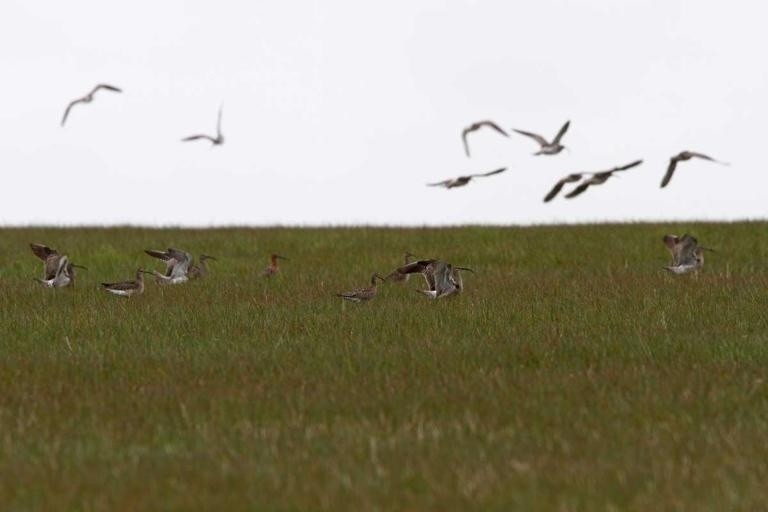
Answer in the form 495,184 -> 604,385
660,151 -> 732,188
99,246 -> 218,302
26,241 -> 89,289
59,82 -> 124,127
256,253 -> 289,285
32,255 -> 71,289
424,118 -> 645,203
179,100 -> 226,147
336,251 -> 475,306
660,231 -> 715,280
